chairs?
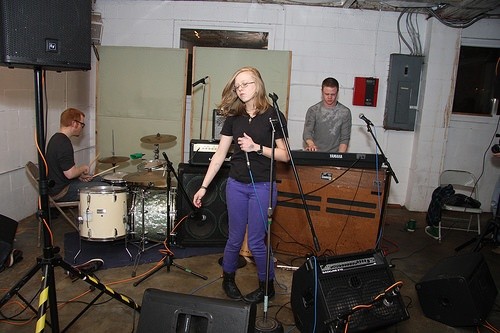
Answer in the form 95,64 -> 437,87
25,161 -> 81,248
425,170 -> 483,242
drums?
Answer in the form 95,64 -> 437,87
130,185 -> 178,247
137,159 -> 167,171
100,171 -> 134,216
77,185 -> 128,242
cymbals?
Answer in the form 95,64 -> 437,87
140,134 -> 177,144
122,171 -> 178,181
97,156 -> 130,163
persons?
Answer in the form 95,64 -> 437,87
194,66 -> 291,303
46,108 -> 111,201
303,78 -> 351,153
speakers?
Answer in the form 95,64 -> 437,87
172,163 -> 230,245
0,0 -> 92,71
290,246 -> 410,333
137,288 -> 257,333
0,213 -> 19,267
416,251 -> 498,327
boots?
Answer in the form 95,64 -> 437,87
242,278 -> 276,304
222,271 -> 242,300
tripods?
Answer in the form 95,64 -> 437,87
0,68 -> 142,333
124,155 -> 165,276
131,168 -> 208,286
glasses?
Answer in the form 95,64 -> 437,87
233,81 -> 256,92
73,119 -> 85,128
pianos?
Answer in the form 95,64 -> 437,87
290,149 -> 383,171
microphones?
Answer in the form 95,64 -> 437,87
359,113 -> 374,126
384,285 -> 401,307
193,76 -> 208,87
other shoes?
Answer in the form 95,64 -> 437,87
8,249 -> 23,267
67,259 -> 104,279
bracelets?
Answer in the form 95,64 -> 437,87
200,186 -> 207,190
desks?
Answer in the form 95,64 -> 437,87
271,166 -> 386,258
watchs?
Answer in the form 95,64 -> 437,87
257,144 -> 263,156
